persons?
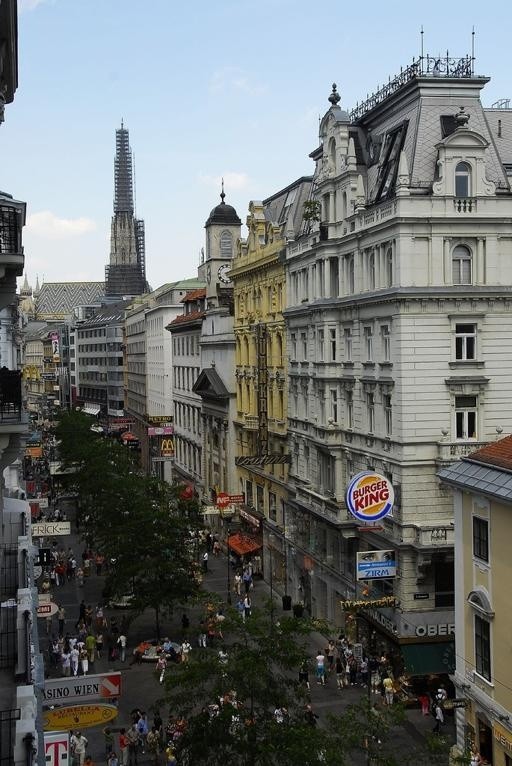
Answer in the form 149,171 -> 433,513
299,628 -> 447,732
37,508 -> 104,594
358,551 -> 392,563
184,528 -> 220,569
470,746 -> 482,766
70,684 -> 288,766
234,569 -> 252,624
46,599 -> 127,677
134,611 -> 229,685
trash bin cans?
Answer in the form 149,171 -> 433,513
283,595 -> 291,610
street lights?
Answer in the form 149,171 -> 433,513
366,627 -> 380,755
226,528 -> 233,605
